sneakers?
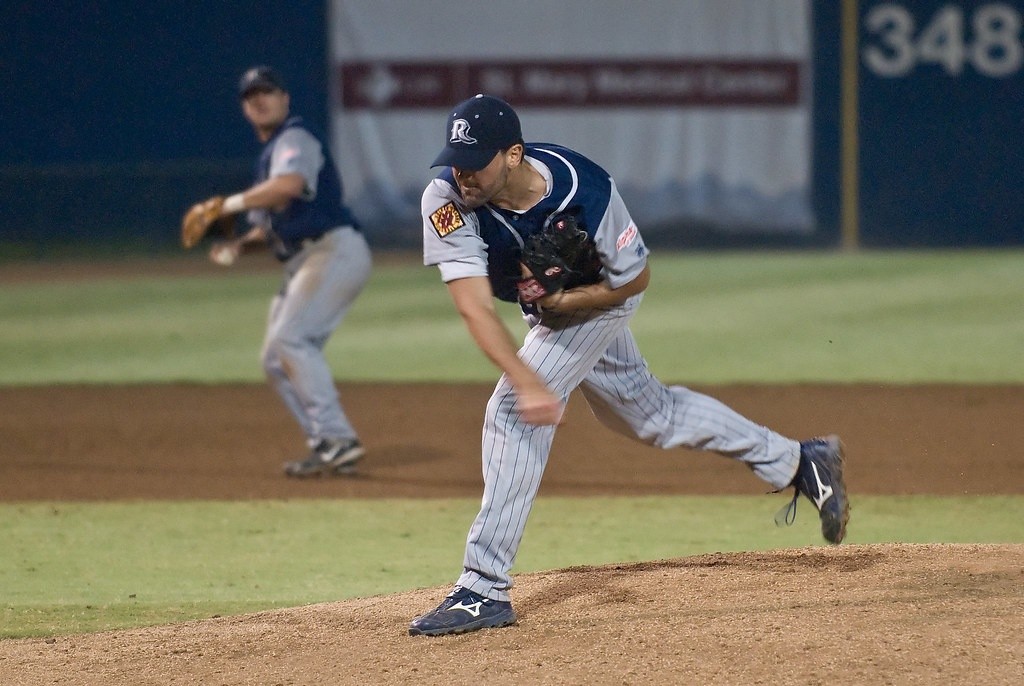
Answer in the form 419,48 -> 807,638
409,586 -> 517,637
283,439 -> 366,475
766,434 -> 849,542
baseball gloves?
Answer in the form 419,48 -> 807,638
515,232 -> 603,321
179,193 -> 237,249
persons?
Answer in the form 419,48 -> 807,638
180,66 -> 374,478
409,95 -> 850,634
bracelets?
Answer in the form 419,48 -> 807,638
222,194 -> 244,212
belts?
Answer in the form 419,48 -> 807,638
293,232 -> 328,252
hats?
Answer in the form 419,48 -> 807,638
239,67 -> 277,98
430,94 -> 522,171
